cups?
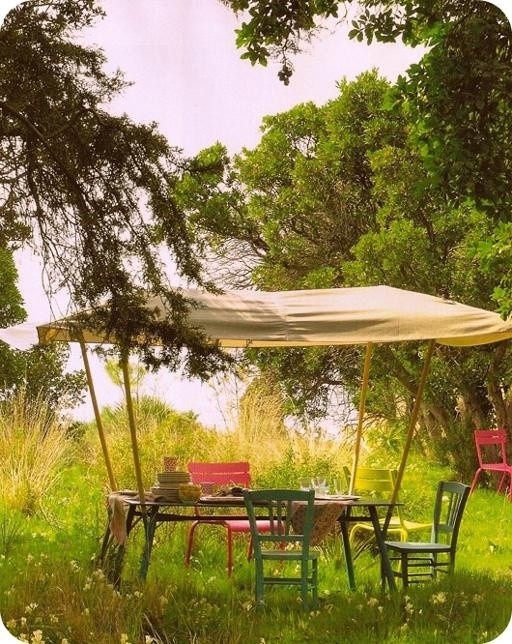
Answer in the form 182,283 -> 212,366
300,474 -> 346,499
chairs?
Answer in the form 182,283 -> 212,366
241,488 -> 319,613
342,465 -> 448,584
380,480 -> 471,596
469,429 -> 512,501
186,462 -> 286,579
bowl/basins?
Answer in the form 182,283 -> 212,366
178,490 -> 202,505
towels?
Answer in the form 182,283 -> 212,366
104,487 -> 169,547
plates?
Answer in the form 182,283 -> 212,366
150,471 -> 191,502
